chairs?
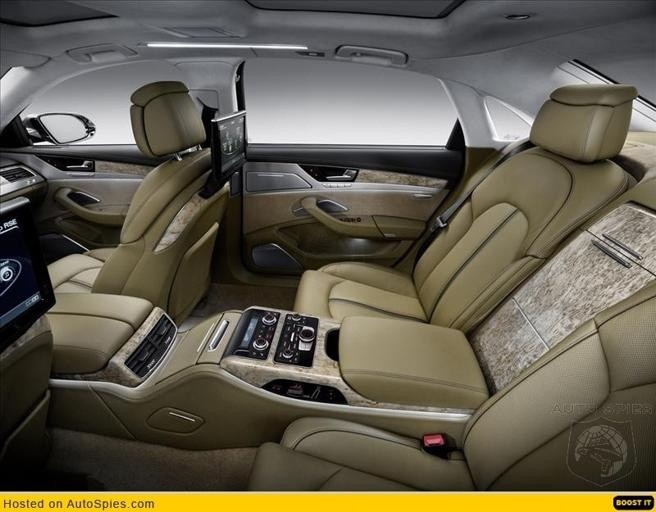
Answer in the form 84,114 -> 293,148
45,79 -> 231,327
0,314 -> 54,475
238,278 -> 656,491
294,84 -> 636,334
